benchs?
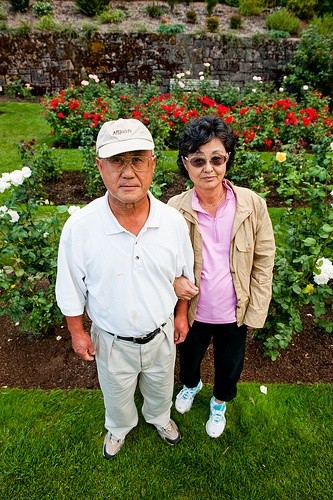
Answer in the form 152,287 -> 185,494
169,78 -> 219,95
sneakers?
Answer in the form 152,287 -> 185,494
103,431 -> 124,461
206,396 -> 226,439
175,379 -> 203,414
157,418 -> 182,446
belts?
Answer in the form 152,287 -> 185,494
106,323 -> 167,344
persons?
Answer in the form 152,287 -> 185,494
56,118 -> 195,460
164,118 -> 276,440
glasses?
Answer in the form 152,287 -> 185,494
105,155 -> 154,172
185,153 -> 229,168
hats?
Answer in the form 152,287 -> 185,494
96,118 -> 155,158
